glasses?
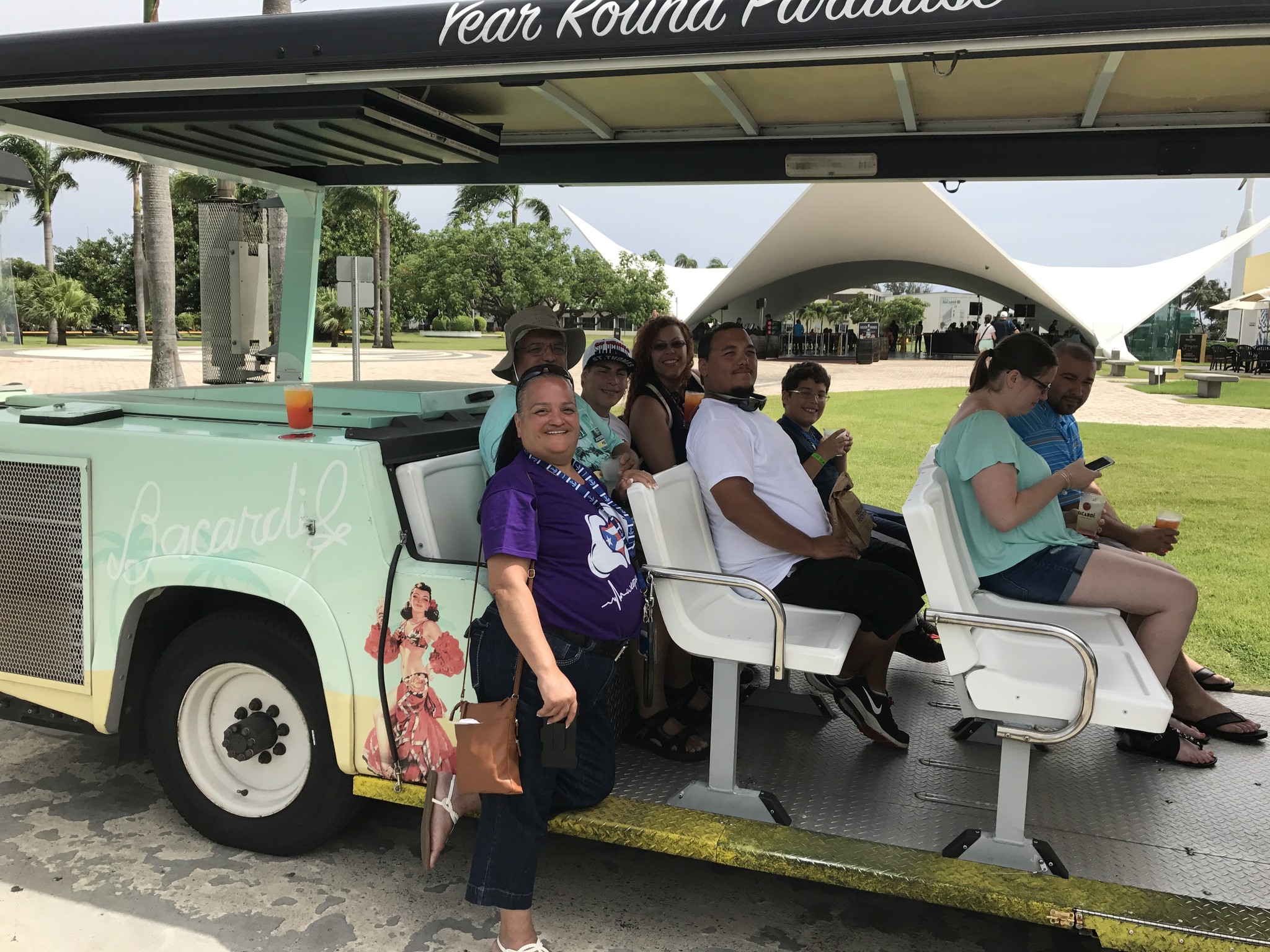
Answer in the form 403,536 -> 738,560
707,390 -> 767,412
516,364 -> 574,410
516,342 -> 567,356
788,389 -> 831,401
650,340 -> 687,350
1006,371 -> 1051,394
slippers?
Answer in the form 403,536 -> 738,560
1171,708 -> 1268,740
1192,667 -> 1234,690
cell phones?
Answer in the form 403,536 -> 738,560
1084,456 -> 1115,471
540,701 -> 578,769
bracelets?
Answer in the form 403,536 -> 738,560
1052,468 -> 1073,496
811,452 -> 826,466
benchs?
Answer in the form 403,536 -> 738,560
901,445 -> 1173,879
1138,364 -> 1178,385
626,461 -> 861,826
1185,372 -> 1239,399
390,448 -> 486,566
1094,356 -> 1108,370
1105,360 -> 1136,375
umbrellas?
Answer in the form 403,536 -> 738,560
1208,287 -> 1270,346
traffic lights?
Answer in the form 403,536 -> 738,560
766,319 -> 772,334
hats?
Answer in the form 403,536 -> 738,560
491,305 -> 586,381
582,337 -> 637,378
1000,311 -> 1008,317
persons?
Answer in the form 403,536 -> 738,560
685,323 -> 926,750
622,314 -> 707,474
774,360 -> 946,663
736,311 -> 1059,358
1008,340 -> 1268,743
421,361 -> 643,952
479,306 -> 712,763
935,329 -> 1217,768
578,337 -> 631,449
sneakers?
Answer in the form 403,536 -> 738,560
894,621 -> 946,663
833,676 -> 909,750
916,610 -> 939,636
805,672 -> 853,695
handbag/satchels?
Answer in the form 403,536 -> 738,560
974,343 -> 980,353
449,697 -> 523,796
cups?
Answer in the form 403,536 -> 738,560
599,454 -> 623,498
823,428 -> 847,457
683,390 -> 705,429
1154,508 -> 1183,553
283,383 -> 313,438
1076,491 -> 1106,537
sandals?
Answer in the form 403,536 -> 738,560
420,770 -> 459,872
1115,713 -> 1211,743
497,935 -> 549,952
627,706 -> 710,763
1116,723 -> 1218,767
664,678 -> 712,725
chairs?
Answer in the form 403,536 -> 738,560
1209,344 -> 1270,375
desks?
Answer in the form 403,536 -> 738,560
1223,348 -> 1265,371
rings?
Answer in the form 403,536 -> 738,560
642,470 -> 645,472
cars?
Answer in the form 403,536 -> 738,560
31,323 -> 45,331
91,320 -> 131,334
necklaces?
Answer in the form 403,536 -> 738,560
608,414 -> 610,425
